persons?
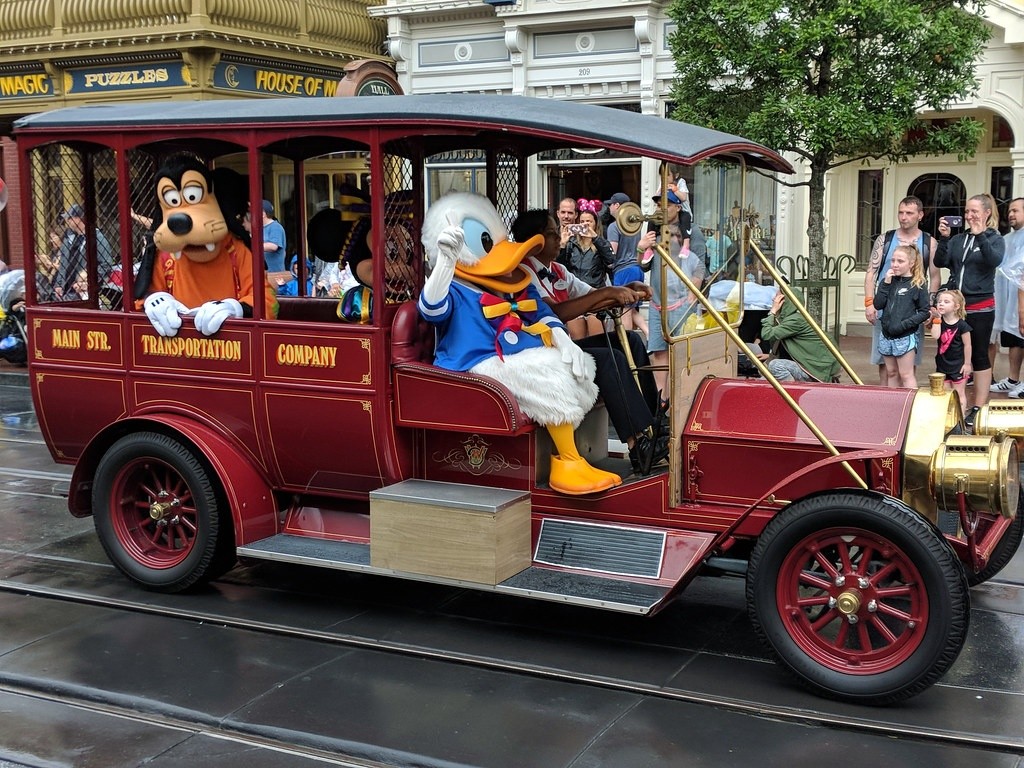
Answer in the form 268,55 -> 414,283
865,194 -> 1024,427
641,162 -> 693,265
10,204 -> 153,331
761,288 -> 842,385
512,209 -> 669,473
636,189 -> 706,414
239,197 -> 360,300
552,193 -> 649,341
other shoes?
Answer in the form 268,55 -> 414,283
641,251 -> 654,265
679,245 -> 690,259
629,435 -> 673,471
654,416 -> 671,438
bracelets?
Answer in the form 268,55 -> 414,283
932,319 -> 942,324
865,297 -> 873,307
636,248 -> 644,254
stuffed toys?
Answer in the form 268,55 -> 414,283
307,186 -> 430,326
416,191 -> 622,496
133,154 -> 279,337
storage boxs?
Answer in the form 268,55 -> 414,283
368,477 -> 532,585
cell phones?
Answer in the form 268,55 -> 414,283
944,216 -> 962,227
567,224 -> 588,236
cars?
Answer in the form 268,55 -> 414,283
13,87 -> 1023,711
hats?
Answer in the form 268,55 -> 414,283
604,193 -> 630,204
61,204 -> 85,216
652,188 -> 681,204
260,199 -> 273,216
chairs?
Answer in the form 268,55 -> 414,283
736,309 -> 772,377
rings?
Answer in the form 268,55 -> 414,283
625,299 -> 629,304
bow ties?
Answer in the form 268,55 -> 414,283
538,267 -> 556,283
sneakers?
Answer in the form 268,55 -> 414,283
990,378 -> 1022,393
964,406 -> 981,426
1008,383 -> 1024,398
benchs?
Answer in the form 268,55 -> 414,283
389,298 -> 604,436
